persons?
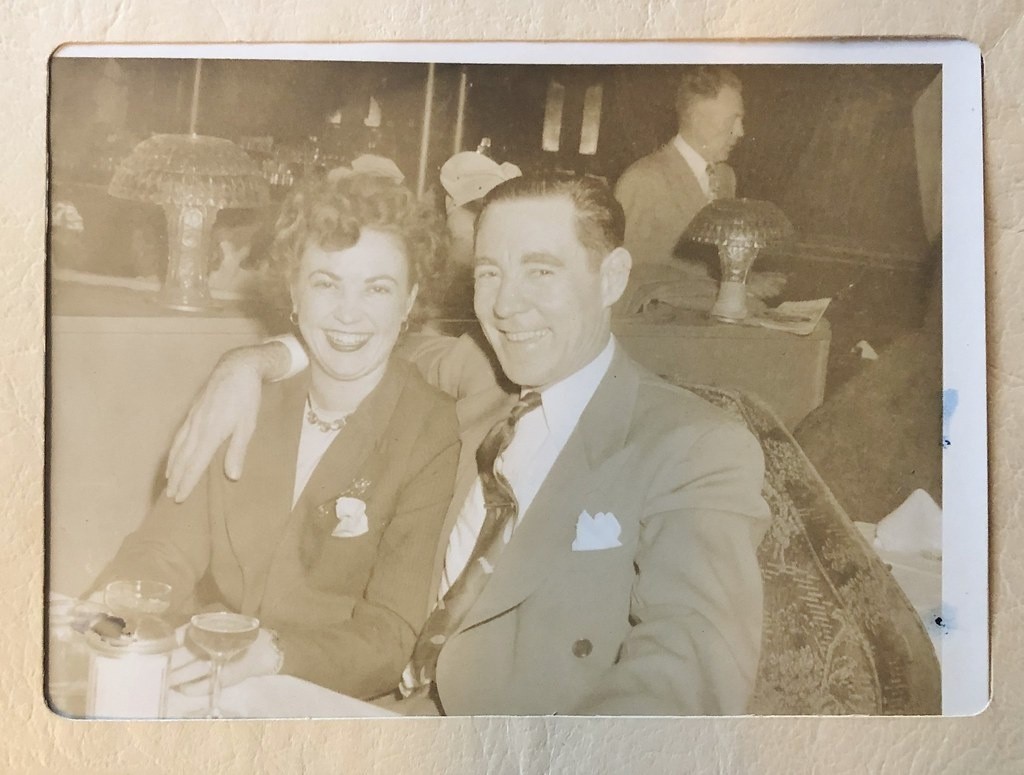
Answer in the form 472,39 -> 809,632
69,171 -> 462,699
164,169 -> 771,715
614,64 -> 789,314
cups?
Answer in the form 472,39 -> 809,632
105,579 -> 172,618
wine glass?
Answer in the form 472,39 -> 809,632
189,612 -> 259,719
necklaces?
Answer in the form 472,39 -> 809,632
304,392 -> 351,431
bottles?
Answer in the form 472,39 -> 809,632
477,137 -> 491,157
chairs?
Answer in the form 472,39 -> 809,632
673,381 -> 944,715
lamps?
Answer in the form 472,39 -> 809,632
111,133 -> 269,310
682,200 -> 793,324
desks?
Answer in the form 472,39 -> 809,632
615,304 -> 832,431
52,279 -> 263,586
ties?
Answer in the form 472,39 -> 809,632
412,392 -> 542,687
707,163 -> 718,200
